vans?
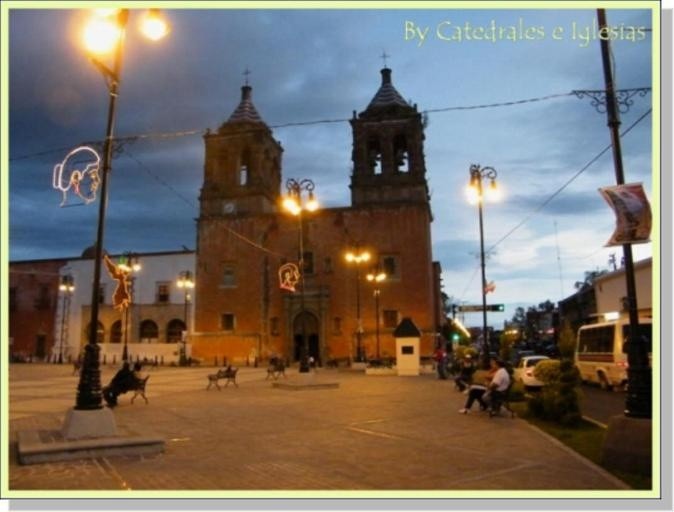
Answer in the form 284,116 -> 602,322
512,355 -> 550,387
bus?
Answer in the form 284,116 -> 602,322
574,319 -> 652,392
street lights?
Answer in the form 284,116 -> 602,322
58,8 -> 171,441
465,163 -> 503,369
176,271 -> 195,365
282,178 -> 318,372
345,241 -> 386,371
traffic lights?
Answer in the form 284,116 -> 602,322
452,332 -> 460,341
492,304 -> 504,311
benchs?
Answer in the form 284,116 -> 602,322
265,366 -> 285,382
96,374 -> 150,409
479,376 -> 516,418
206,368 -> 239,391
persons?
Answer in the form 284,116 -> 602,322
102,360 -> 130,405
458,360 -> 514,414
481,356 -> 510,411
131,362 -> 143,379
